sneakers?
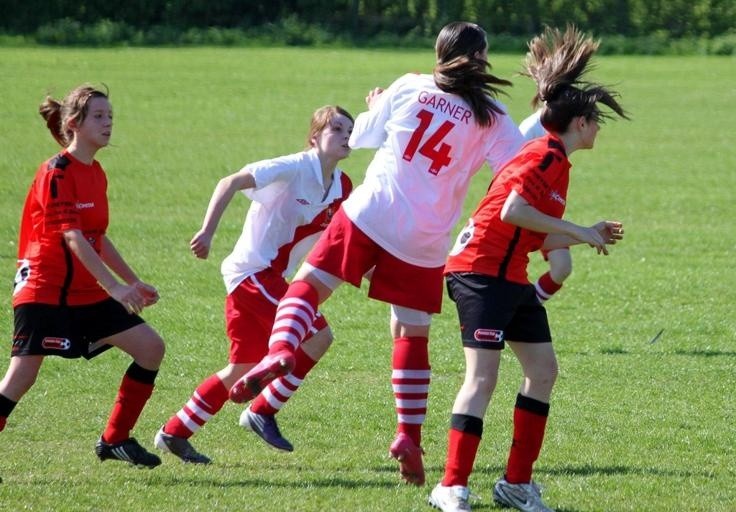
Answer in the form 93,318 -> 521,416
389,432 -> 425,486
96,434 -> 161,469
493,475 -> 552,512
154,426 -> 212,466
228,350 -> 295,404
239,405 -> 293,452
429,483 -> 472,512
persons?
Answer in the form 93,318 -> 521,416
231,21 -> 527,486
0,81 -> 164,467
425,26 -> 625,511
515,23 -> 602,305
154,106 -> 356,464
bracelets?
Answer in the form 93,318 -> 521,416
107,280 -> 117,293
